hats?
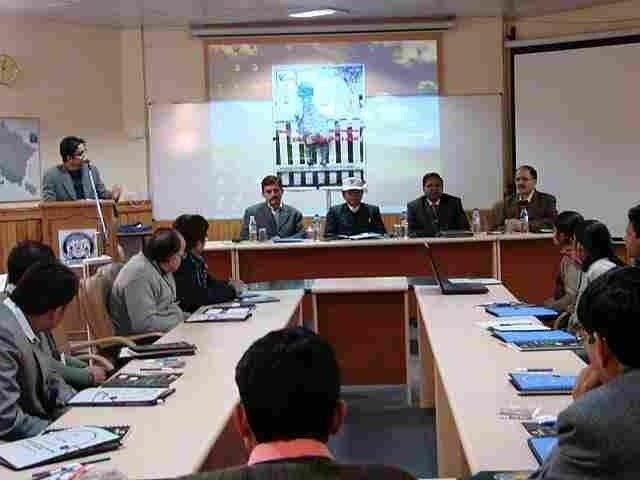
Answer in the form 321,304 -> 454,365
340,176 -> 368,193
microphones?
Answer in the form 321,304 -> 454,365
80,158 -> 90,164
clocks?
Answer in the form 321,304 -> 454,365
0,54 -> 21,84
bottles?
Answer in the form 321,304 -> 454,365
399,208 -> 409,239
248,215 -> 258,241
312,214 -> 322,240
520,208 -> 529,237
471,207 -> 481,233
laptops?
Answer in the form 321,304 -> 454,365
430,255 -> 489,294
440,230 -> 473,237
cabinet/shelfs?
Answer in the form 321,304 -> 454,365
303,275 -> 411,387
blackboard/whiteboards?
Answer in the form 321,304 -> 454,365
150,95 -> 501,220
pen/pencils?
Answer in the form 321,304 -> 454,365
499,322 -> 533,327
32,468 -> 65,480
273,238 -> 305,244
514,366 -> 556,373
138,367 -> 174,372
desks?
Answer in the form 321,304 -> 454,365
235,236 -> 497,280
0,288 -> 308,479
201,239 -> 235,280
413,278 -> 590,480
497,232 -> 631,306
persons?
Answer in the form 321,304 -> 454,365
40,134 -> 125,203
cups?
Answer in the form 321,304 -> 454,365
392,223 -> 402,240
